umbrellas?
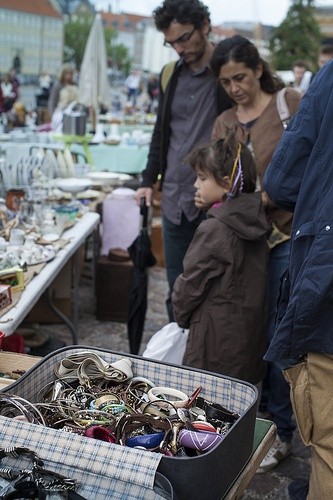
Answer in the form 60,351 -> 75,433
78,13 -> 110,131
127,198 -> 157,354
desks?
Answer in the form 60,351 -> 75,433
0,112 -> 155,174
1,212 -> 101,345
225,418 -> 278,500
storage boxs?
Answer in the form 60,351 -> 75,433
0,346 -> 259,500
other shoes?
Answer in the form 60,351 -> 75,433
288,478 -> 308,500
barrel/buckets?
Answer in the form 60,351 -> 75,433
63,102 -> 87,135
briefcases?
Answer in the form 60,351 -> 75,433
0,345 -> 259,500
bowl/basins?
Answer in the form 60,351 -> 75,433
57,178 -> 91,192
90,171 -> 131,186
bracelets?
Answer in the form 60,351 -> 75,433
0,352 -> 241,451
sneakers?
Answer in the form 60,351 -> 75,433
257,433 -> 291,473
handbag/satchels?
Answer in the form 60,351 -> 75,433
142,321 -> 190,365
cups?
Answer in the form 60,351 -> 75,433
0,201 -> 79,269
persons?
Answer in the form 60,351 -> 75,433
262,60 -> 333,500
48,64 -> 77,113
0,68 -> 20,111
283,36 -> 333,94
124,64 -> 159,101
211,34 -> 296,473
135,0 -> 235,323
172,122 -> 275,385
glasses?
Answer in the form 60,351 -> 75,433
163,27 -> 198,48
